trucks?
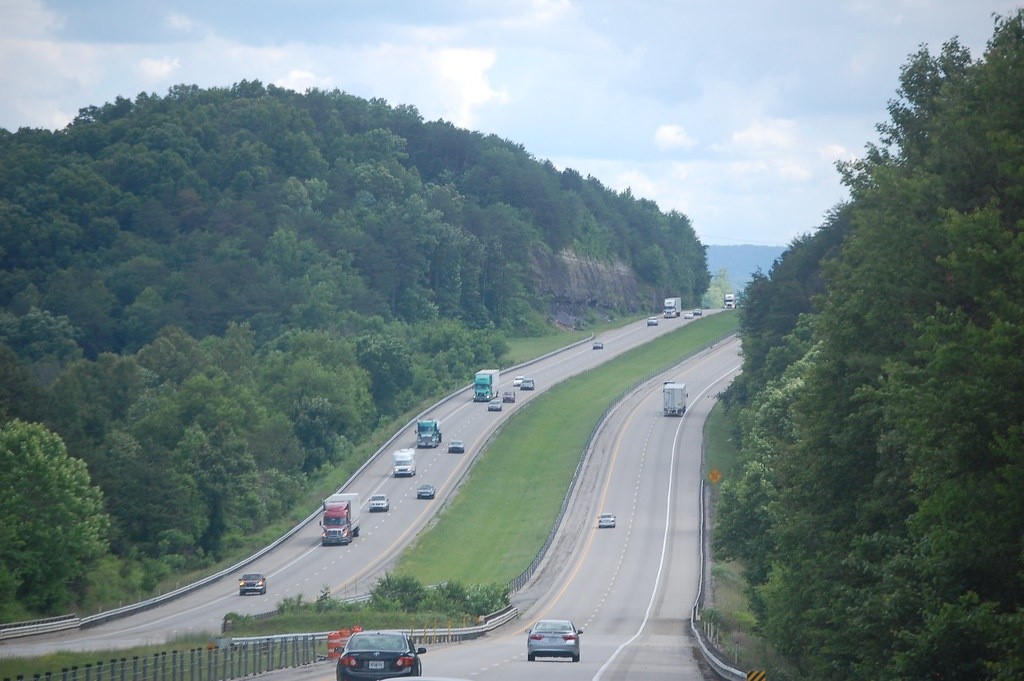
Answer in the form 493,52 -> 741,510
393,449 -> 416,477
663,298 -> 682,318
415,419 -> 441,449
473,370 -> 499,403
319,493 -> 361,546
725,294 -> 736,309
663,383 -> 688,416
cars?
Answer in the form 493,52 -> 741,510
448,440 -> 465,453
514,376 -> 526,387
684,312 -> 693,319
526,620 -> 584,662
593,342 -> 603,349
417,484 -> 436,500
238,574 -> 267,596
647,317 -> 658,326
489,400 -> 502,411
597,513 -> 616,529
368,495 -> 390,513
334,631 -> 426,681
693,309 -> 702,316
521,378 -> 535,390
503,392 -> 516,403
